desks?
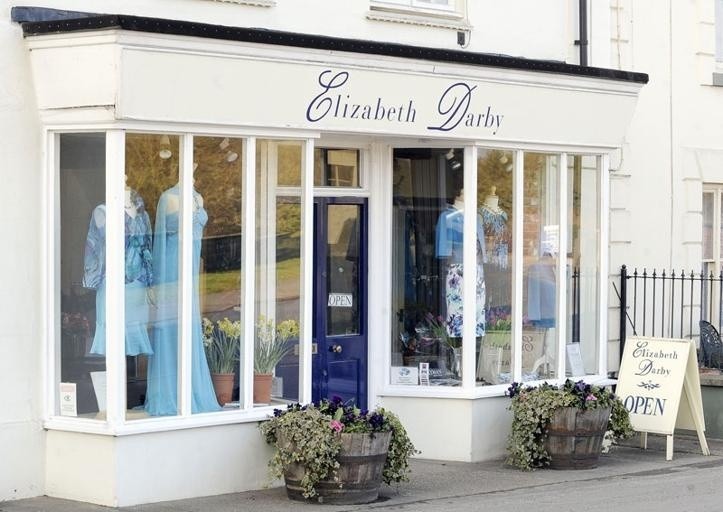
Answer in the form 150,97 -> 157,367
61,352 -> 105,414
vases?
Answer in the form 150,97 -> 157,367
452,346 -> 462,381
253,374 -> 274,404
211,374 -> 235,406
485,348 -> 503,386
71,334 -> 86,360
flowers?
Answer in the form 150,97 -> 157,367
255,400 -> 421,501
485,306 -> 529,348
61,310 -> 91,336
253,314 -> 300,376
415,312 -> 454,350
200,315 -> 241,374
502,377 -> 637,473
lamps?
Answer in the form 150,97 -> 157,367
216,138 -> 238,163
443,147 -> 462,171
498,152 -> 514,173
158,135 -> 173,161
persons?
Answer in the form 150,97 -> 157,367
525,251 -> 561,330
79,184 -> 155,360
471,187 -> 511,332
141,171 -> 223,416
433,189 -> 486,339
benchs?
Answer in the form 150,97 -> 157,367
201,231 -> 292,273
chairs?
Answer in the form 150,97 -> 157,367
327,217 -> 357,259
698,320 -> 723,370
